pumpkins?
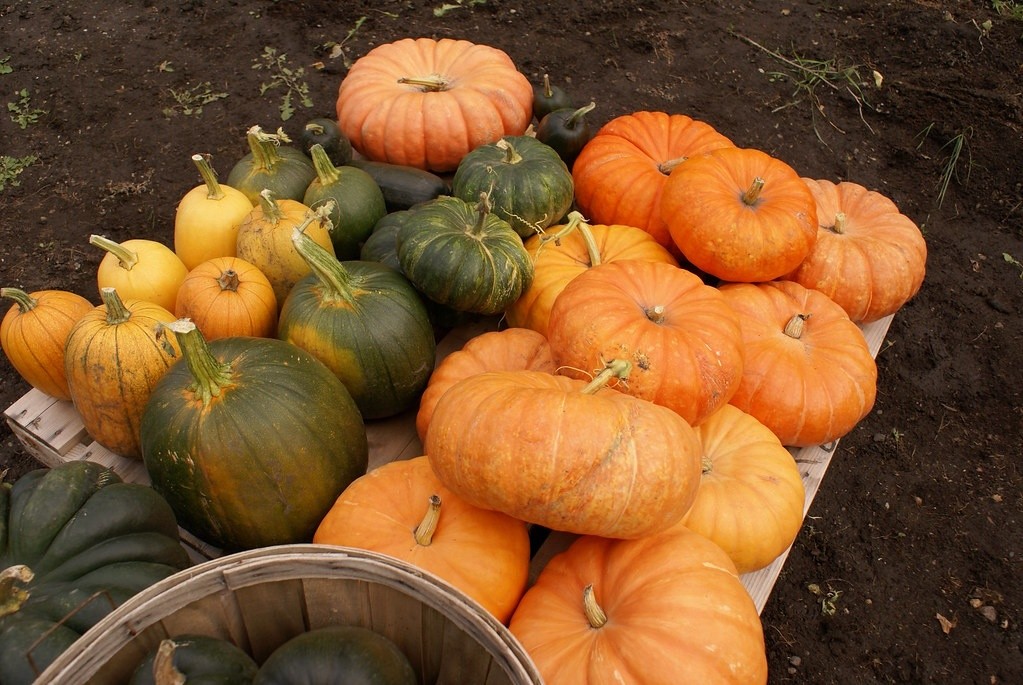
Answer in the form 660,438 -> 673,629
534,73 -> 574,123
425,357 -> 700,539
130,625 -> 416,685
547,260 -> 744,426
783,177 -> 926,323
336,38 -> 535,169
573,111 -> 739,258
681,403 -> 806,572
314,455 -> 529,625
0,460 -> 193,685
535,102 -> 595,174
453,135 -> 575,236
397,192 -> 534,314
716,280 -> 877,448
661,147 -> 819,280
0,117 -> 455,550
505,211 -> 680,338
415,328 -> 561,447
509,523 -> 767,685
